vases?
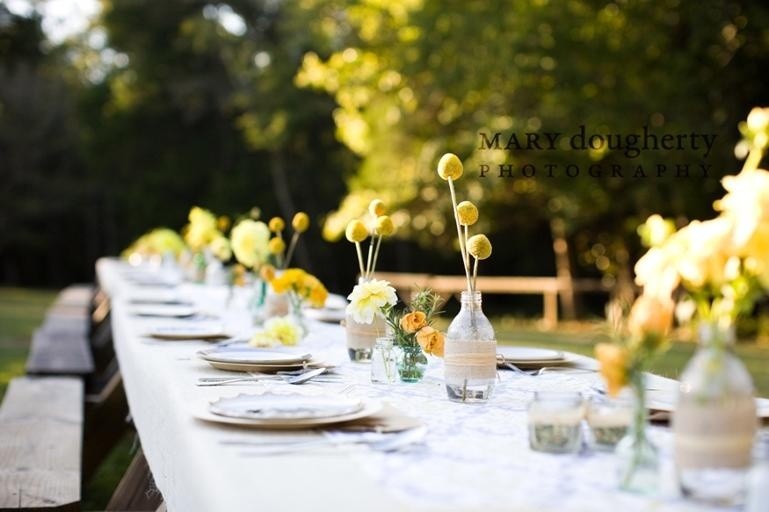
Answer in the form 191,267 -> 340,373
345,299 -> 387,356
612,423 -> 669,494
374,336 -> 400,382
398,342 -> 424,382
673,339 -> 761,500
444,288 -> 498,407
284,310 -> 312,338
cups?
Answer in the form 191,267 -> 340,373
588,397 -> 637,451
526,397 -> 585,460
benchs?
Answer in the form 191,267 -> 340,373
40,301 -> 87,334
0,374 -> 85,509
57,283 -> 93,304
22,322 -> 95,373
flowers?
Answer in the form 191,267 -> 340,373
382,280 -> 447,377
631,103 -> 768,338
268,268 -> 330,310
347,199 -> 394,280
348,280 -> 396,374
232,217 -> 275,273
268,212 -> 311,270
438,152 -> 491,397
595,254 -> 661,471
119,206 -> 232,261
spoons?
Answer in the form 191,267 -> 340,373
194,366 -> 329,388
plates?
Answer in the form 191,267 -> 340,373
177,382 -> 388,431
494,346 -> 583,368
194,346 -> 327,372
